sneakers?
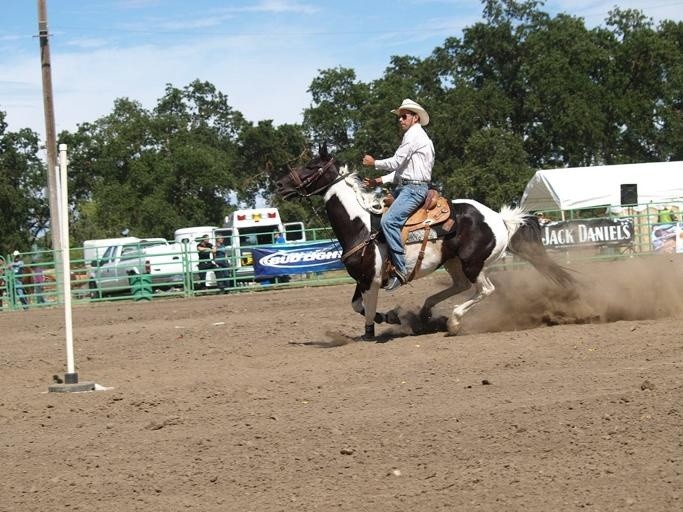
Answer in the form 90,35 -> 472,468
385,276 -> 400,290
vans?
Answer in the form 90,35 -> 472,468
212,207 -> 306,286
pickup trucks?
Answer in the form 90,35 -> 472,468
87,237 -> 199,298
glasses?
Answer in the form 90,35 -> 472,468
401,114 -> 407,119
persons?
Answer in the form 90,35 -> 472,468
657,209 -> 678,223
358,96 -> 436,292
29,261 -> 51,308
6,250 -> 28,311
0,255 -> 6,311
271,228 -> 289,283
195,235 -> 219,297
208,234 -> 232,296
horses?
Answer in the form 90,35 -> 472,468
274,140 -> 583,340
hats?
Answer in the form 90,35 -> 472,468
11,250 -> 23,259
389,98 -> 430,126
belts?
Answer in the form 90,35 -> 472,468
400,179 -> 422,184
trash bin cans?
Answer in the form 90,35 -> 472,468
129,272 -> 152,301
